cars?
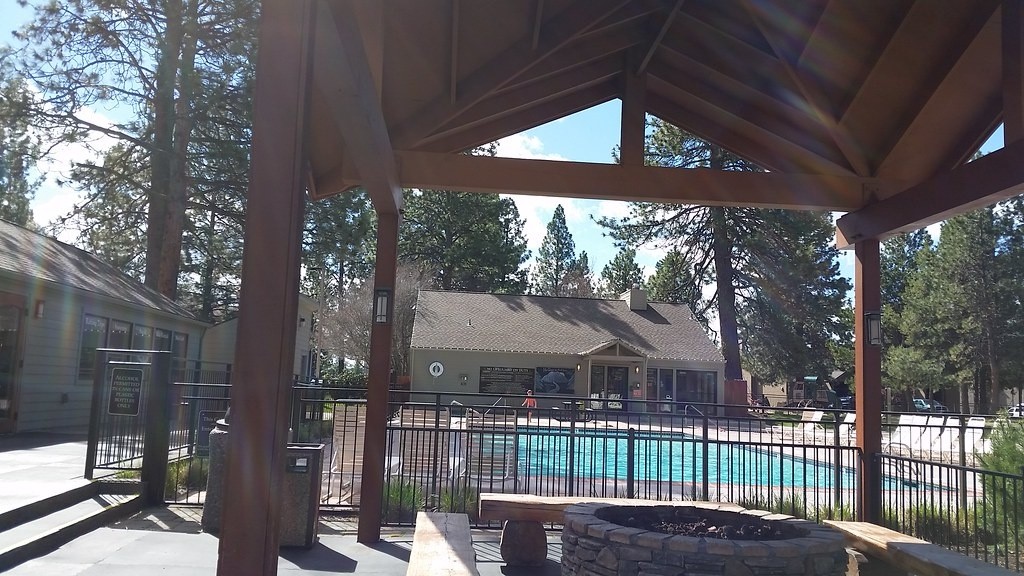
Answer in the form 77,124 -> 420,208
996,403 -> 1024,418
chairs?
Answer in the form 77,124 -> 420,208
401,402 -> 466,508
321,399 -> 401,502
454,404 -> 524,495
765,408 -> 1011,465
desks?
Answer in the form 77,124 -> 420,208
563,402 -> 584,421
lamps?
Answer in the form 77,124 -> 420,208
864,310 -> 882,347
631,382 -> 641,391
35,299 -> 46,319
372,287 -> 392,325
459,373 -> 468,385
577,365 -> 581,372
635,366 -> 640,373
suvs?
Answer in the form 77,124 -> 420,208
828,396 -> 855,409
914,399 -> 948,413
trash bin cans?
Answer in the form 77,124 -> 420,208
280,442 -> 326,547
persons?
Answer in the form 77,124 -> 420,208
521,390 -> 537,422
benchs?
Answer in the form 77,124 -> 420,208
585,408 -> 593,422
552,406 -> 561,420
406,512 -> 480,576
824,520 -> 1024,576
479,493 -> 748,566
